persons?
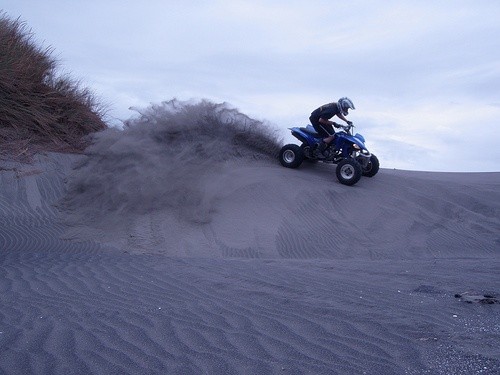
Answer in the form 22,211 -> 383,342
309,96 -> 355,159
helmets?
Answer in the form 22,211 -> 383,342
337,97 -> 356,116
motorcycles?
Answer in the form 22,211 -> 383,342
278,121 -> 380,185
